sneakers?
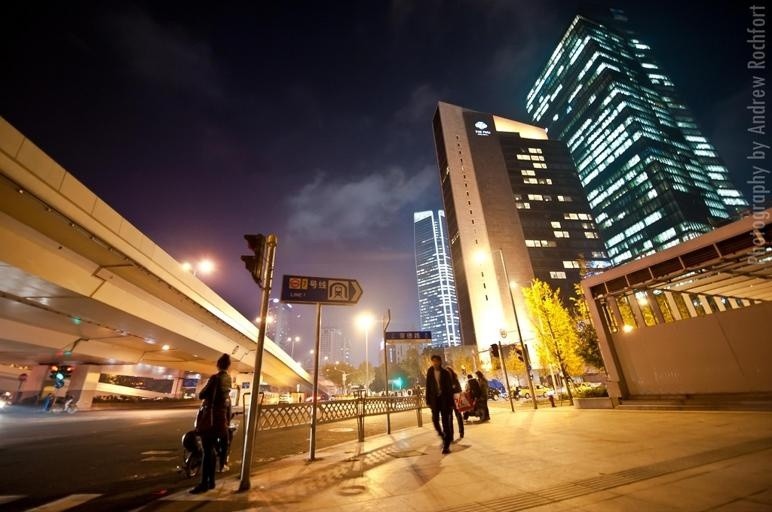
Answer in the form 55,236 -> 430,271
205,480 -> 216,489
188,483 -> 208,494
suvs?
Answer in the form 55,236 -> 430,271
280,393 -> 292,404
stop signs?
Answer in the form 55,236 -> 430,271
18,373 -> 28,382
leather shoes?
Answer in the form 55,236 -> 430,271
442,445 -> 449,453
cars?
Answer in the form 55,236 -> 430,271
486,384 -> 501,402
510,382 -> 600,399
0,391 -> 17,407
378,386 -> 426,398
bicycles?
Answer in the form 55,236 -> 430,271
503,388 -> 522,402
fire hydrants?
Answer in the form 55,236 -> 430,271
547,393 -> 557,408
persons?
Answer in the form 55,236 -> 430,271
42,393 -> 75,414
425,355 -> 454,453
445,366 -> 464,442
464,370 -> 490,424
188,354 -> 233,495
513,387 -> 521,400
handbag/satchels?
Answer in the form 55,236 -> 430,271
454,391 -> 475,413
193,404 -> 213,429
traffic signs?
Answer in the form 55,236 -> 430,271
278,273 -> 365,307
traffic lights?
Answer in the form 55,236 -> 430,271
47,364 -> 58,379
237,231 -> 267,285
490,343 -> 499,358
515,348 -> 524,363
54,372 -> 64,389
61,365 -> 72,379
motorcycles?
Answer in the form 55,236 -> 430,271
176,411 -> 244,481
51,404 -> 78,415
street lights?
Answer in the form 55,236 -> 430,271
286,335 -> 301,359
180,258 -> 218,277
352,309 -> 378,385
255,315 -> 273,335
471,247 -> 539,410
